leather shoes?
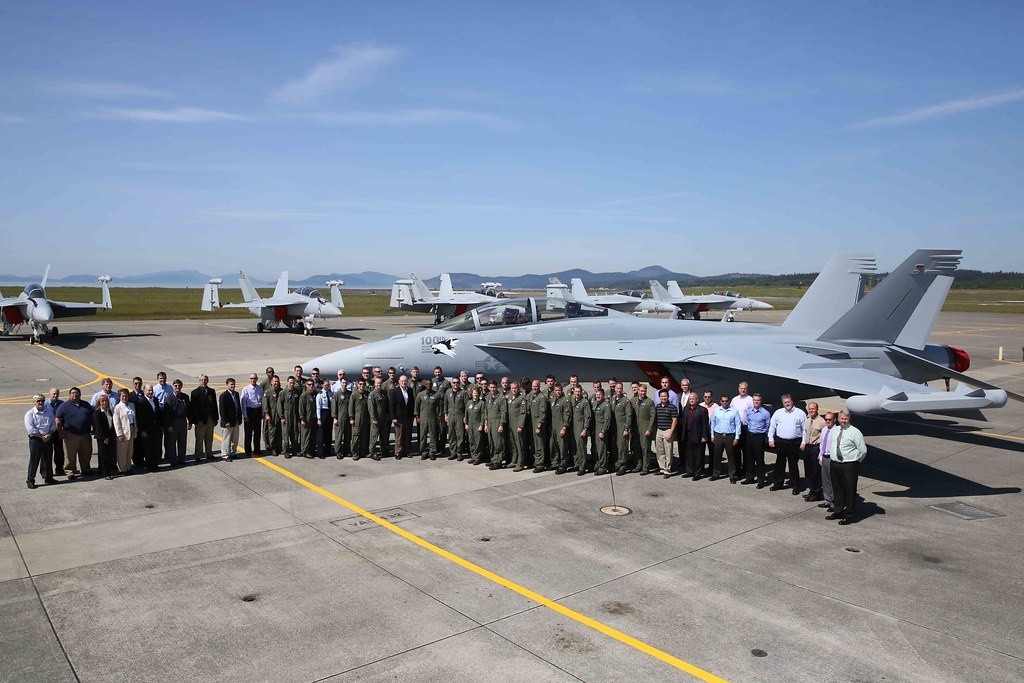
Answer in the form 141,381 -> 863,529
682,471 -> 849,525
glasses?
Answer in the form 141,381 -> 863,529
375,371 -> 382,373
312,373 -> 319,375
721,400 -> 728,402
133,383 -> 141,385
174,385 -> 181,387
452,382 -> 460,384
476,377 -> 483,379
250,378 -> 257,380
358,383 -> 365,385
34,400 -> 45,402
338,374 -> 345,376
703,396 -> 711,398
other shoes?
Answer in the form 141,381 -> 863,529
24,451 -> 669,489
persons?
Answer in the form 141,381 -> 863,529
24,372 -> 219,489
219,377 -> 243,462
817,412 -> 837,513
825,410 -> 868,525
240,365 -> 771,489
802,402 -> 826,503
768,393 -> 807,495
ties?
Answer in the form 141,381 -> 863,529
809,418 -> 814,444
682,394 -> 685,406
837,427 -> 843,461
822,429 -> 830,457
326,392 -> 331,410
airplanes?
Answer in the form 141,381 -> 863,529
385,271 -> 526,326
0,263 -> 114,345
544,275 -> 682,319
200,268 -> 345,336
300,246 -> 1024,415
647,280 -> 774,322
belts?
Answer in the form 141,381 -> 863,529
823,454 -> 830,458
